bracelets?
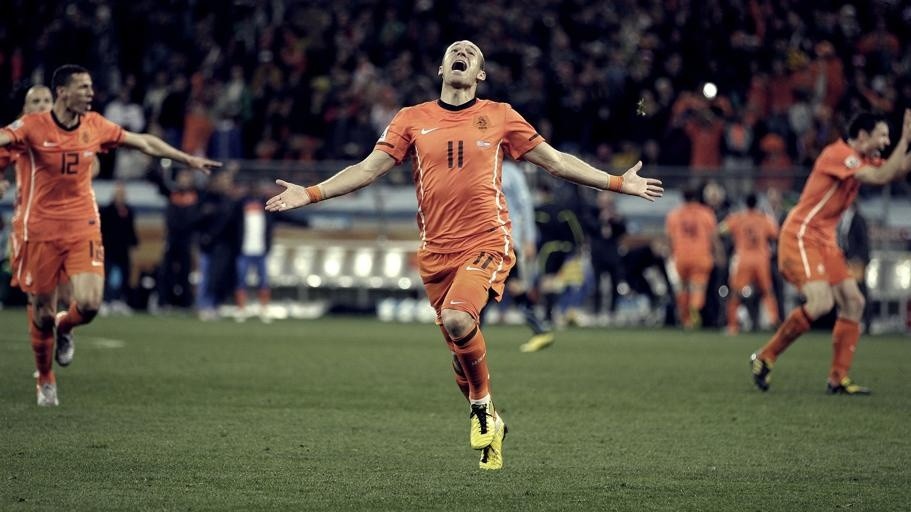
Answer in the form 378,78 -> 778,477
305,185 -> 323,203
608,174 -> 625,192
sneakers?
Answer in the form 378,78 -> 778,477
478,418 -> 508,470
53,311 -> 73,366
35,382 -> 58,408
519,332 -> 554,354
825,378 -> 870,396
469,399 -> 495,450
748,352 -> 773,392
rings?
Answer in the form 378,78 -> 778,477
282,202 -> 287,208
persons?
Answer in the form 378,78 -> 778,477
0,0 -> 910,191
261,37 -> 666,473
746,110 -> 911,396
500,155 -> 874,354
0,64 -> 225,406
97,169 -> 311,321
